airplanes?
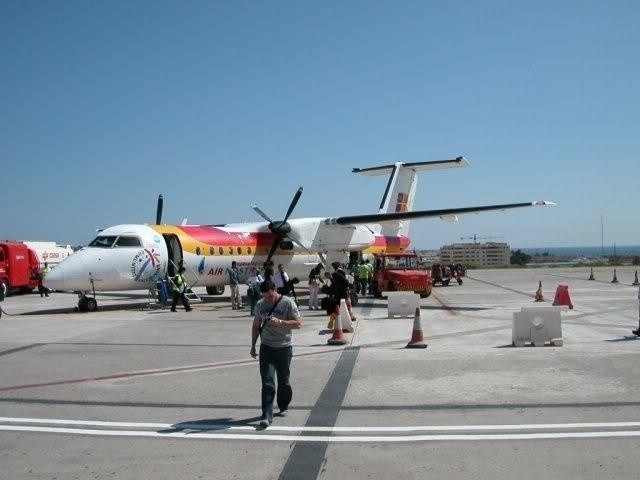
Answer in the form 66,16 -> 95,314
42,157 -> 557,311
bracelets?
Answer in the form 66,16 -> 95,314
279,319 -> 282,327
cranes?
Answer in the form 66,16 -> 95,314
460,234 -> 505,243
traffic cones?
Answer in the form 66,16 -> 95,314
408,307 -> 427,346
346,298 -> 356,321
535,281 -> 545,302
611,269 -> 619,283
589,267 -> 595,280
632,269 -> 640,285
328,306 -> 346,345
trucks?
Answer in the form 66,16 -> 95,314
373,252 -> 432,297
0,240 -> 83,297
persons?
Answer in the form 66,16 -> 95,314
259,274 -> 278,297
171,267 -> 194,312
308,263 -> 326,310
357,261 -> 371,296
274,264 -> 290,294
246,269 -> 265,315
330,262 -> 350,308
365,259 -> 374,290
250,283 -> 302,427
350,260 -> 361,294
228,261 -> 245,310
38,262 -> 51,297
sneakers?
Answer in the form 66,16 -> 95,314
260,418 -> 270,428
278,409 -> 289,418
